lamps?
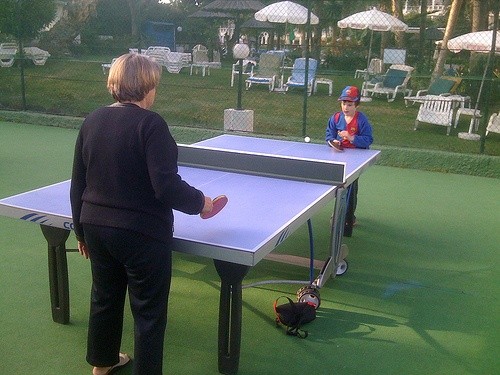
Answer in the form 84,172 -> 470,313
234,43 -> 249,60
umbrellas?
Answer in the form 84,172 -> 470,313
188,0 -> 275,59
254,1 -> 320,89
337,7 -> 408,72
447,29 -> 499,135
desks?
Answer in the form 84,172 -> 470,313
314,78 -> 334,96
454,107 -> 481,131
448,96 -> 472,109
361,81 -> 375,95
0,134 -> 381,375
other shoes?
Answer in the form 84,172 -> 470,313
344,223 -> 353,236
346,215 -> 356,223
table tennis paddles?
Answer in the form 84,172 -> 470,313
328,140 -> 344,152
200,195 -> 228,219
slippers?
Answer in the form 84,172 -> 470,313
92,353 -> 130,375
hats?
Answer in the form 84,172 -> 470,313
338,86 -> 361,102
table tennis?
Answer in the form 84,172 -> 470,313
305,137 -> 310,143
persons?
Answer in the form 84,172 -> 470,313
325,85 -> 373,237
69,53 -> 213,374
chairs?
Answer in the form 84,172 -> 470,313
231,48 -> 317,96
414,97 -> 453,135
102,45 -> 221,76
485,111 -> 500,137
0,43 -> 51,67
356,58 -> 462,108
